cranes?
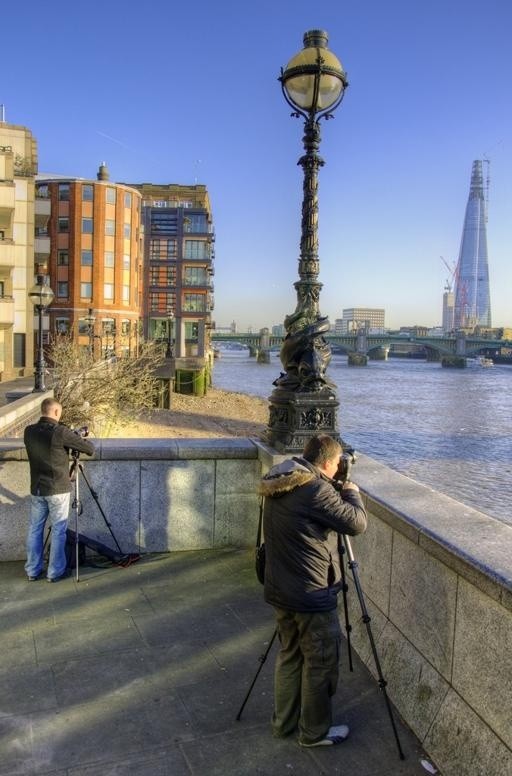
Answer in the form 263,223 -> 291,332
439,255 -> 469,329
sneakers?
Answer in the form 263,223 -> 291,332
28,568 -> 71,583
298,725 -> 349,748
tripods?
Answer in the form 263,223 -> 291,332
43,448 -> 124,582
236,481 -> 403,759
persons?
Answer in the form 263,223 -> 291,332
255,433 -> 367,746
24,398 -> 94,583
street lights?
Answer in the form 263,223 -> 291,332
263,24 -> 350,451
85,305 -> 98,369
165,306 -> 177,359
26,274 -> 55,396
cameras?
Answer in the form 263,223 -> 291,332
73,426 -> 89,438
334,449 -> 356,480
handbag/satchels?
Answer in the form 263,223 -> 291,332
255,542 -> 265,586
